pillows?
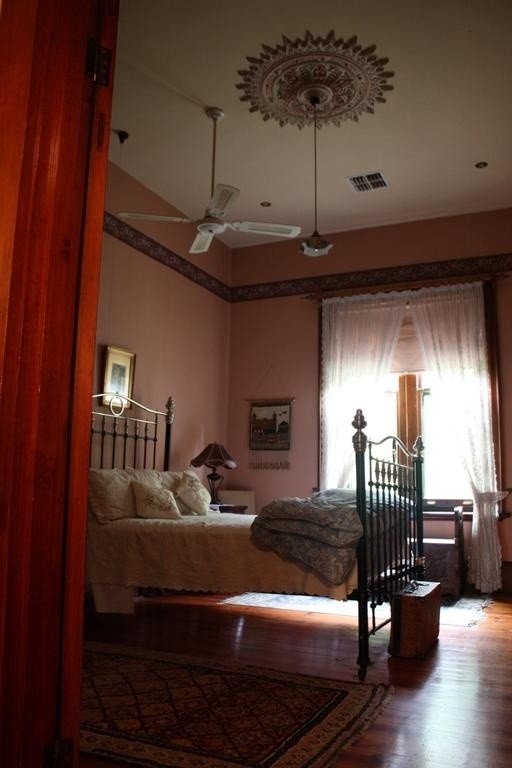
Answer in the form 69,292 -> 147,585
88,465 -> 212,521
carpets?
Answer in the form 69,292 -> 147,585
78,632 -> 397,768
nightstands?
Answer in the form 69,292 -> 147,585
210,504 -> 248,514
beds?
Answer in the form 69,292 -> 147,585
85,394 -> 423,688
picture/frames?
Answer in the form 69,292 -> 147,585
101,346 -> 137,411
246,397 -> 293,453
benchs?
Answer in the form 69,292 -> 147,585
406,506 -> 466,604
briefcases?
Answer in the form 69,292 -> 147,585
388,580 -> 442,659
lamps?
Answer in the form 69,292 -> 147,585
188,441 -> 238,505
299,91 -> 334,258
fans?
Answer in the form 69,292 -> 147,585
115,106 -> 303,256
